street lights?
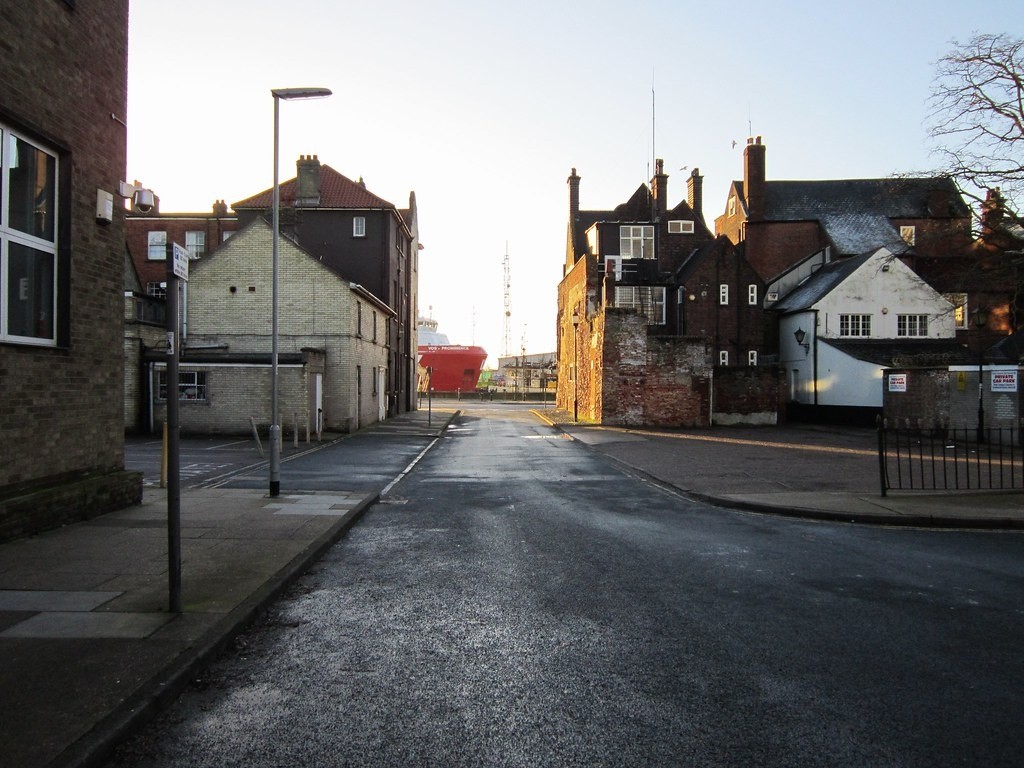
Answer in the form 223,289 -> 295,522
269,85 -> 333,502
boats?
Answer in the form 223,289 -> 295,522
417,305 -> 488,391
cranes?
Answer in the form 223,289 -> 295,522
501,237 -> 513,357
